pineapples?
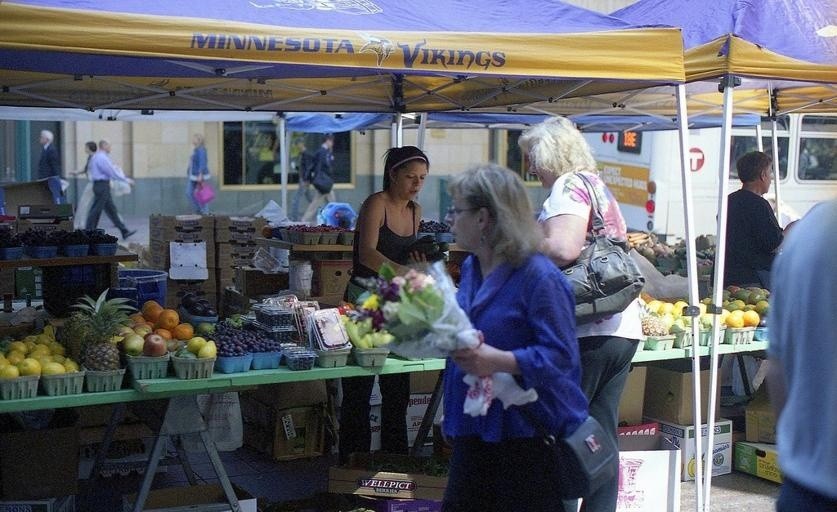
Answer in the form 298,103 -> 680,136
642,317 -> 668,336
69,324 -> 100,366
84,342 -> 120,371
56,312 -> 91,349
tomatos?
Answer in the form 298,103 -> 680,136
286,224 -> 355,232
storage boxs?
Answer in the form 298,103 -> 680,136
122,480 -> 258,512
312,257 -> 353,297
328,462 -> 449,500
614,360 -> 785,512
331,406 -> 381,454
0,426 -> 80,501
410,371 -> 441,394
336,378 -> 383,407
149,213 -> 288,320
405,394 -> 444,448
258,381 -> 328,409
0,491 -> 76,512
1,175 -> 74,234
241,392 -> 325,462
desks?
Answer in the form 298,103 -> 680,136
0,384 -> 255,512
0,245 -> 138,288
128,356 -> 447,512
631,341 -> 769,396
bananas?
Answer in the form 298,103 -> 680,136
627,231 -> 649,249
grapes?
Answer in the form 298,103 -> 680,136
419,219 -> 451,233
209,323 -> 282,358
1,225 -> 118,251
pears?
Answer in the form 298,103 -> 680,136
1,333 -> 80,380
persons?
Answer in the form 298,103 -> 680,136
517,116 -> 641,512
260,138 -> 281,183
36,130 -> 63,197
300,133 -> 337,223
710,152 -> 787,292
438,164 -> 588,512
338,146 -> 430,463
185,133 -> 210,215
69,142 -> 132,232
765,197 -> 837,512
290,138 -> 315,221
85,140 -> 137,239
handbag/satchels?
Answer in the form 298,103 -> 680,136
194,181 -> 215,204
558,234 -> 646,326
540,415 -> 618,501
313,171 -> 332,194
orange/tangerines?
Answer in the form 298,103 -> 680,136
700,284 -> 771,314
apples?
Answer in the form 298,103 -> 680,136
135,324 -> 154,336
141,300 -> 158,313
117,325 -> 136,333
143,306 -> 161,324
159,309 -> 180,329
130,315 -> 144,321
145,322 -> 156,328
175,322 -> 194,340
143,334 -> 168,357
154,328 -> 173,339
123,334 -> 145,356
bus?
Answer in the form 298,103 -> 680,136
578,113 -> 837,247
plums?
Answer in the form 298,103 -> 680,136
182,293 -> 198,308
191,303 -> 206,315
199,300 -> 210,308
205,307 -> 218,317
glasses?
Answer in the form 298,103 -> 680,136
445,205 -> 479,215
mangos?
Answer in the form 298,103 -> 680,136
188,337 -> 206,352
199,340 -> 218,358
